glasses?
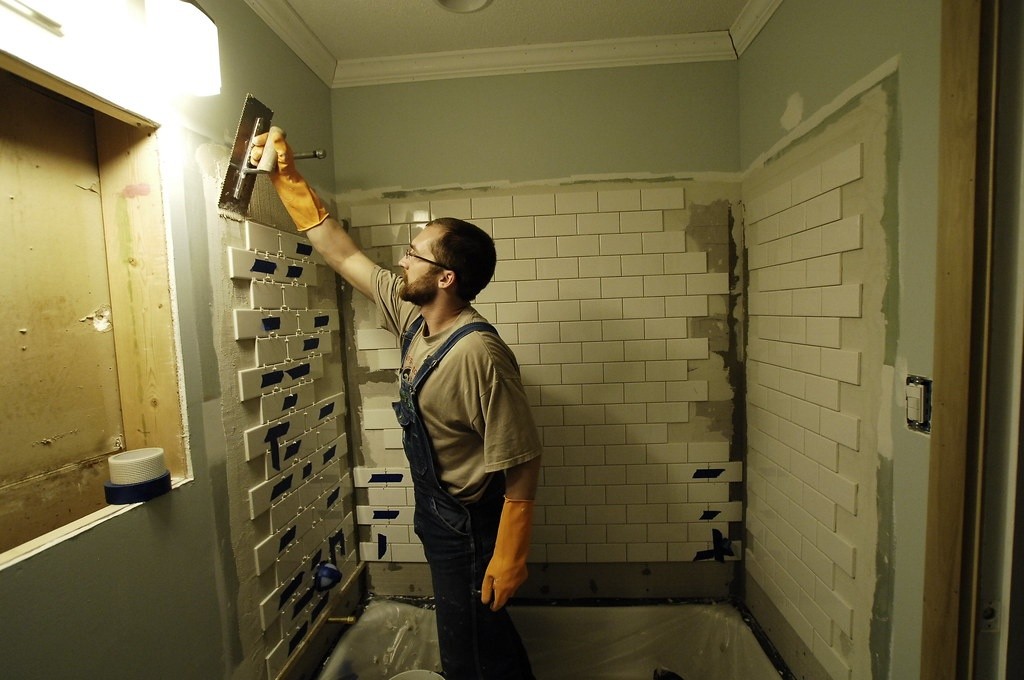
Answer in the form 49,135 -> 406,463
405,248 -> 452,270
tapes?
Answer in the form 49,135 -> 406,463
108,448 -> 167,484
106,469 -> 172,505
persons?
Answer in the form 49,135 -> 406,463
250,132 -> 543,680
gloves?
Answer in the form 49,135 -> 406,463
250,131 -> 329,232
481,495 -> 536,612
